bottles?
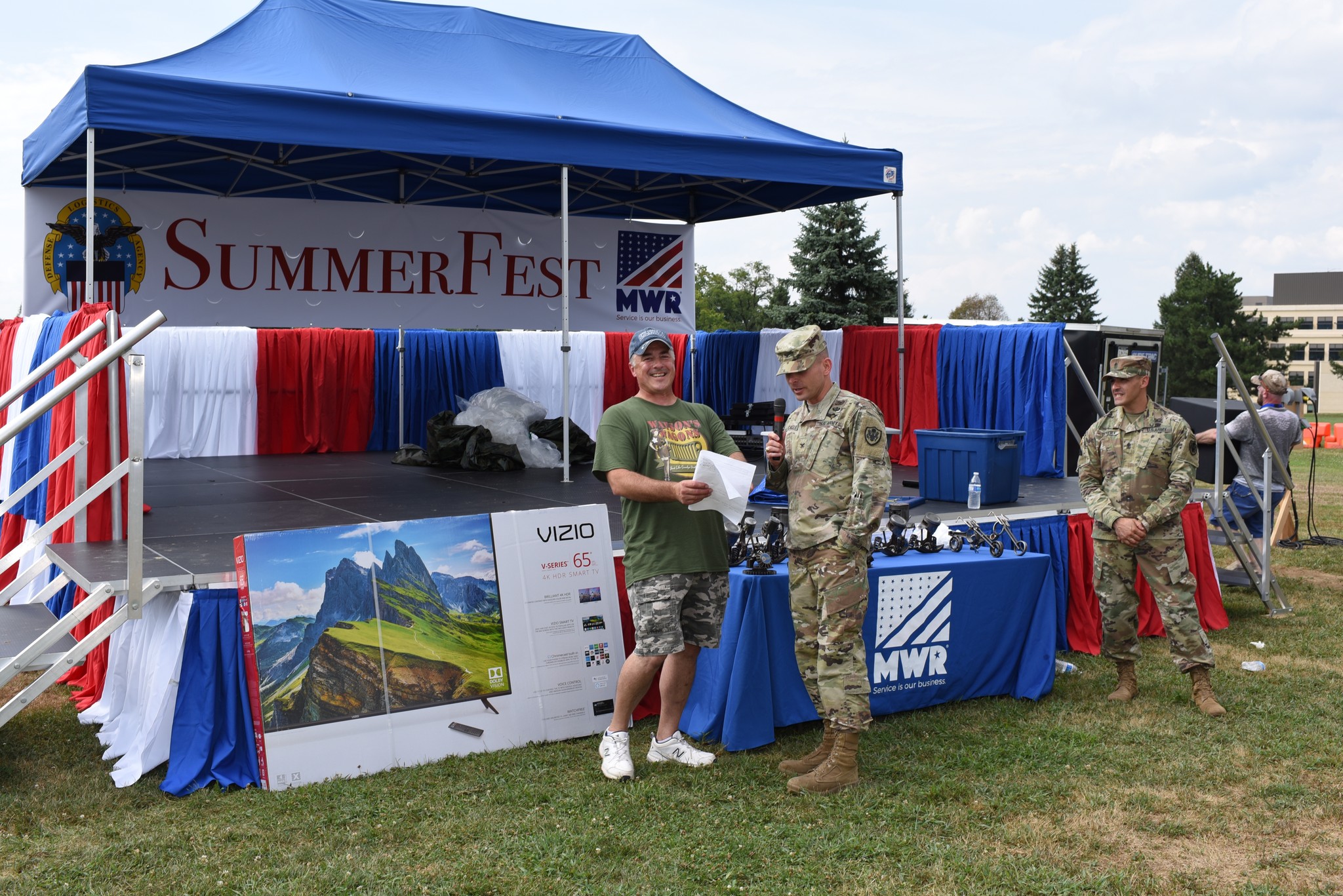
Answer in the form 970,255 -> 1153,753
968,472 -> 981,509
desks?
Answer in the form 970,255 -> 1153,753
676,546 -> 1056,751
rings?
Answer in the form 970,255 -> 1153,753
765,446 -> 769,452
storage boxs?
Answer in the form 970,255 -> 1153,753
914,428 -> 1026,504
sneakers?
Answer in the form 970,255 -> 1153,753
598,728 -> 635,781
647,730 -> 718,766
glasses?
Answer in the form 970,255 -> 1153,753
1258,372 -> 1269,391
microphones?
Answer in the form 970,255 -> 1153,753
772,398 -> 786,461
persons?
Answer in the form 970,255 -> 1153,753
1075,356 -> 1227,717
1193,368 -> 1301,588
592,328 -> 753,783
764,324 -> 892,797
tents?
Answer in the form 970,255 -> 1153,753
21,0 -> 905,486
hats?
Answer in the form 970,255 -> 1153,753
629,327 -> 673,360
1250,369 -> 1288,395
1100,356 -> 1151,381
774,324 -> 828,376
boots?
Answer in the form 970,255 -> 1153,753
779,720 -> 835,776
785,732 -> 859,795
1108,664 -> 1138,702
1190,667 -> 1227,716
